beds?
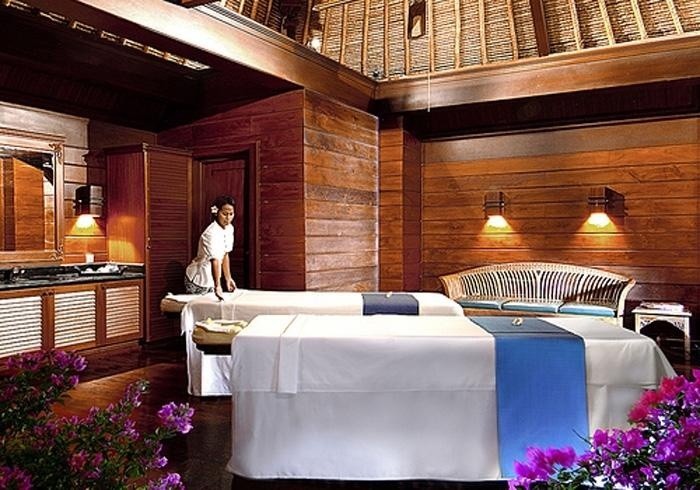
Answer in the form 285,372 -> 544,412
225,313 -> 678,490
162,288 -> 464,397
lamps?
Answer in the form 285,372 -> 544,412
77,185 -> 100,230
482,191 -> 506,230
586,190 -> 612,228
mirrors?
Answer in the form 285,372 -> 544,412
0,127 -> 65,268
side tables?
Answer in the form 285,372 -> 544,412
630,301 -> 692,358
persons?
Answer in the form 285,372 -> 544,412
183,196 -> 239,300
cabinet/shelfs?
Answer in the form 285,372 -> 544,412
0,278 -> 145,359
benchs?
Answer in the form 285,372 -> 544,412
439,262 -> 635,323
